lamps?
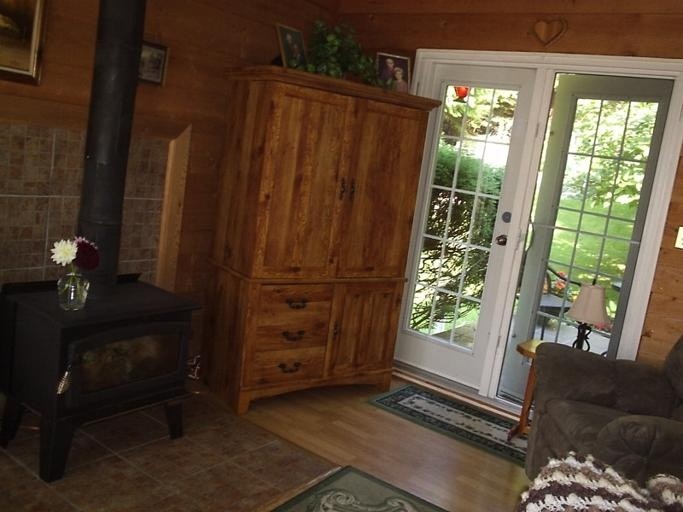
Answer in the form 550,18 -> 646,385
567,283 -> 612,352
450,85 -> 473,105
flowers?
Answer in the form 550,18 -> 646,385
48,236 -> 100,297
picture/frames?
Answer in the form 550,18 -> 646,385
276,23 -> 309,72
375,52 -> 412,97
0,1 -> 51,88
136,41 -> 169,89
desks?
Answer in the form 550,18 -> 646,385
1,280 -> 204,484
505,339 -> 547,438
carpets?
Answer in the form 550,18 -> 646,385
364,381 -> 529,467
267,462 -> 457,511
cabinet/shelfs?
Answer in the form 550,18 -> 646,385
198,63 -> 443,416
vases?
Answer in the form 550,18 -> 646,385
56,273 -> 92,313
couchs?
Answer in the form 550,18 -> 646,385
523,334 -> 682,489
